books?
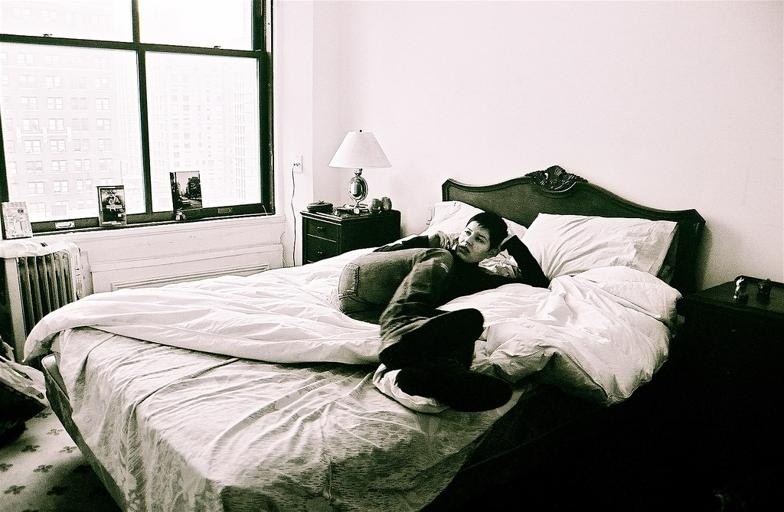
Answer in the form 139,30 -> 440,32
101,188 -> 125,225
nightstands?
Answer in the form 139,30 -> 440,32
677,275 -> 783,469
297,204 -> 398,264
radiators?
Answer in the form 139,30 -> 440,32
16,238 -> 80,341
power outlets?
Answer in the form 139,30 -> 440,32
288,151 -> 305,175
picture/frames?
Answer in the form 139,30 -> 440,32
94,183 -> 128,228
167,168 -> 203,220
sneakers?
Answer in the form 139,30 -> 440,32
395,361 -> 514,413
378,308 -> 483,371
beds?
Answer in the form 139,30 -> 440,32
37,166 -> 711,511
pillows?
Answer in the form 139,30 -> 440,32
428,200 -> 680,276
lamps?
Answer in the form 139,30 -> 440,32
327,129 -> 388,218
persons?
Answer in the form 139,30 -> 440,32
335,209 -> 549,413
